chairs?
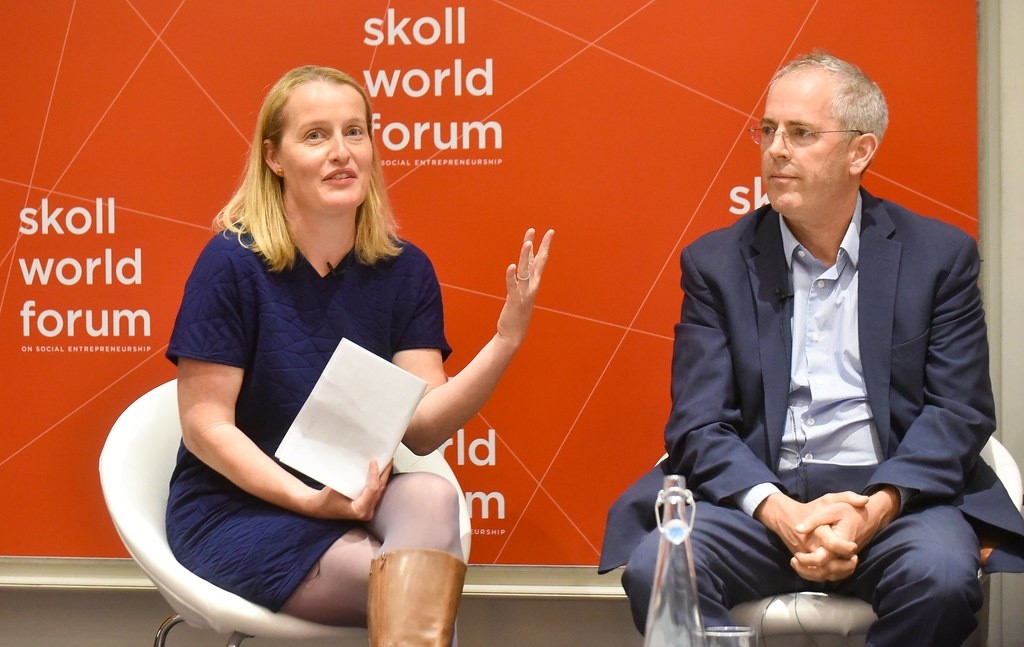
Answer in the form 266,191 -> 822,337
98,378 -> 471,647
728,436 -> 1023,638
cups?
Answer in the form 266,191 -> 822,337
707,626 -> 758,647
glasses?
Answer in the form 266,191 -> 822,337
746,124 -> 864,147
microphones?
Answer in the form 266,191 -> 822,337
775,287 -> 794,302
327,262 -> 345,278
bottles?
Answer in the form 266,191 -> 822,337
642,476 -> 710,647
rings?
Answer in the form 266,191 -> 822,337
516,274 -> 531,281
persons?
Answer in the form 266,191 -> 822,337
166,64 -> 555,646
596,47 -> 1024,646
